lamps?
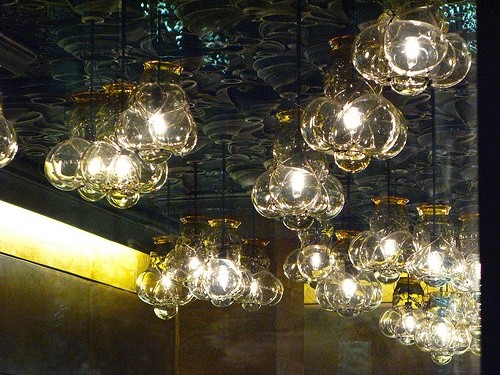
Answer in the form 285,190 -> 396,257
0,0 -> 483,366
